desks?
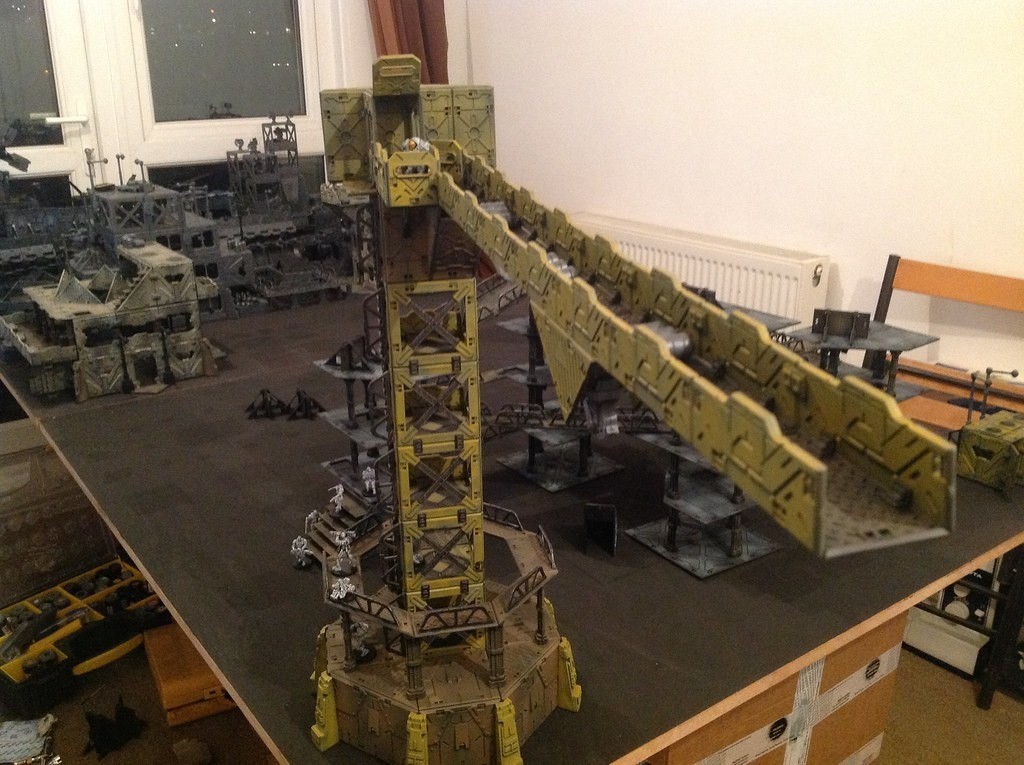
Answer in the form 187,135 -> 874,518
0,292 -> 1024,765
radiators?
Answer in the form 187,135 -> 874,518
571,212 -> 831,352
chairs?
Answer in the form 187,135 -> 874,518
863,255 -> 1024,432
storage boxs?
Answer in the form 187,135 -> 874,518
636,608 -> 911,763
143,624 -> 240,728
